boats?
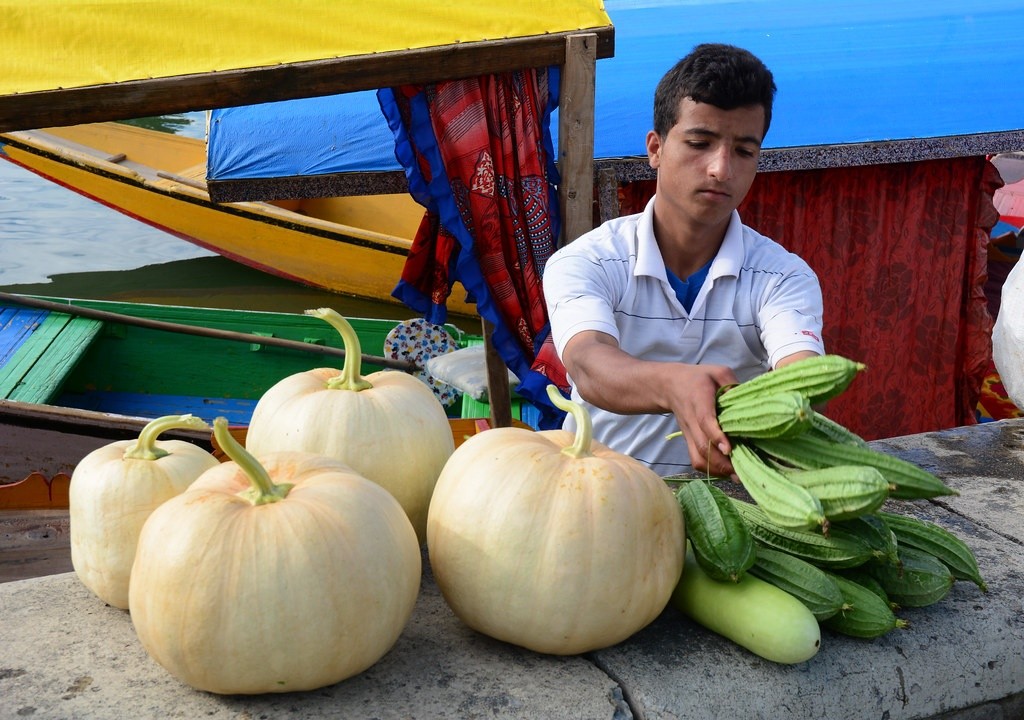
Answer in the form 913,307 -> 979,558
1,292 -> 551,516
0,113 -> 507,326
0,1 -> 1024,458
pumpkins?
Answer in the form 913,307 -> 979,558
242,305 -> 454,547
68,415 -> 226,610
427,386 -> 685,656
128,418 -> 424,694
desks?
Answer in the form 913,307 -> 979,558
0,415 -> 1024,720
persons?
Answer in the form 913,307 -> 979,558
543,43 -> 827,488
